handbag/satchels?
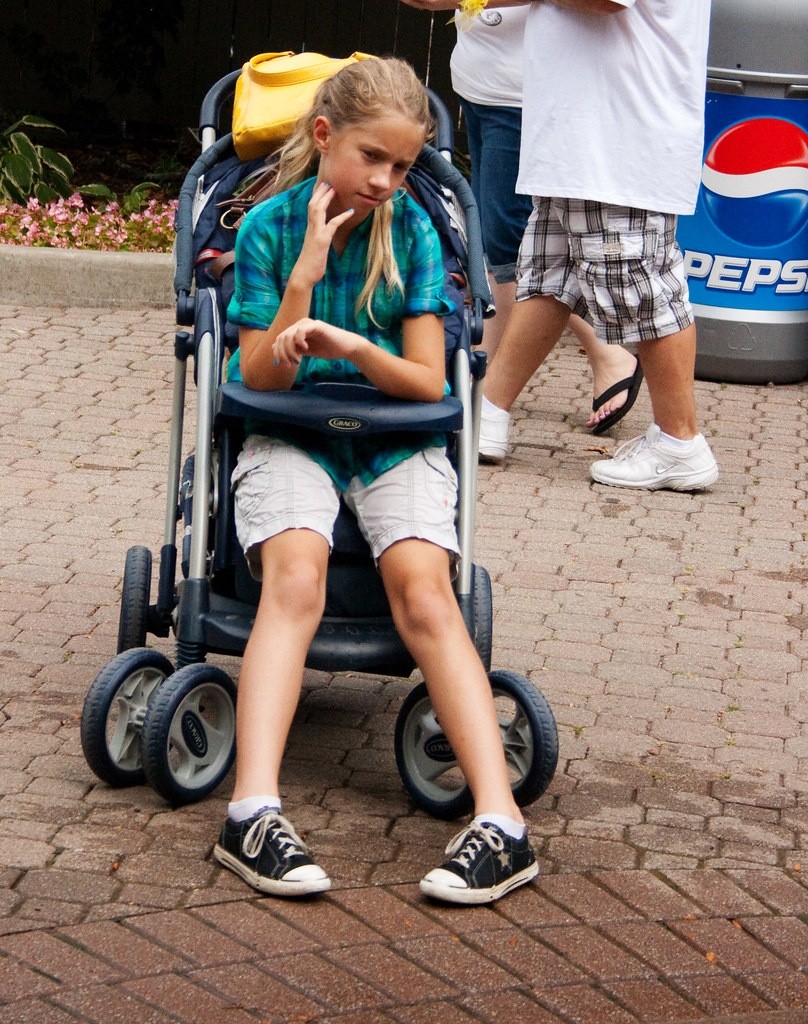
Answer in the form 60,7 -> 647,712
230,48 -> 385,163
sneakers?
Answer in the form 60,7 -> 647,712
213,806 -> 332,895
590,423 -> 720,493
475,409 -> 512,463
420,822 -> 539,905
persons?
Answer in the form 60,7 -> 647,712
402,0 -> 720,492
213,58 -> 540,903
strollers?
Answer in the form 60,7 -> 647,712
80,69 -> 559,821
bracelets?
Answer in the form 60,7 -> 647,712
447,0 -> 487,28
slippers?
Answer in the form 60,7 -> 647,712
590,354 -> 644,432
